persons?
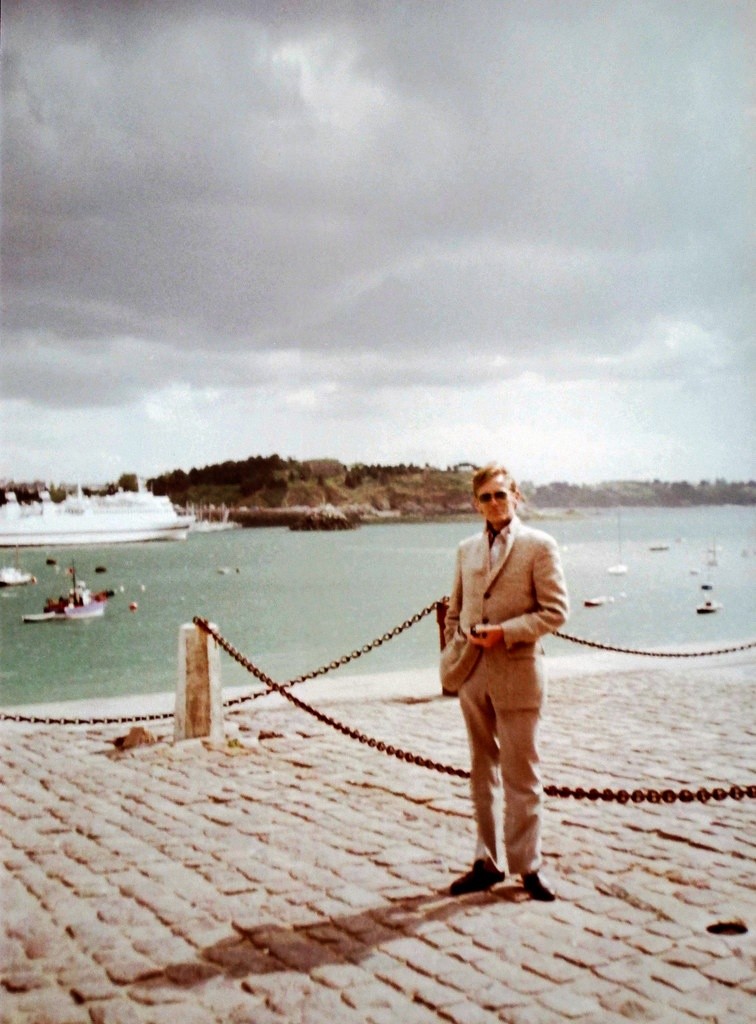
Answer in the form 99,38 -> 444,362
438,468 -> 569,900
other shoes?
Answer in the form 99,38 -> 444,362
450,870 -> 505,896
522,869 -> 556,901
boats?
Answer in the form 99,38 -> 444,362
697,602 -> 717,613
584,599 -> 602,605
23,557 -> 104,622
0,545 -> 31,584
0,486 -> 195,545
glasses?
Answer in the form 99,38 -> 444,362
476,491 -> 510,503
